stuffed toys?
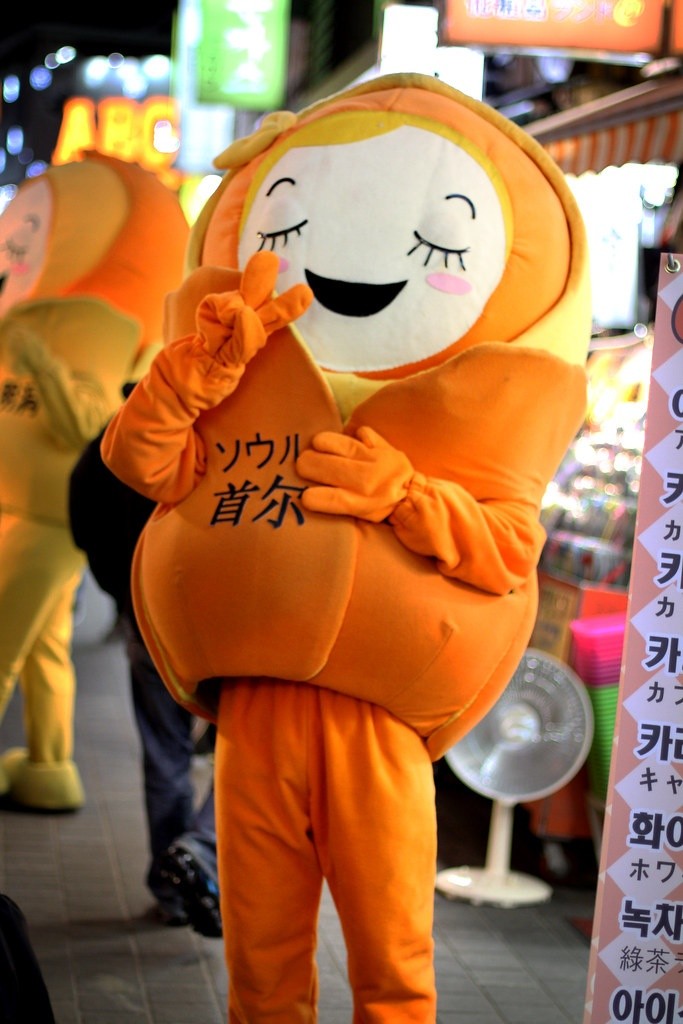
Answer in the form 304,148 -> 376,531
100,71 -> 593,1023
0,148 -> 190,812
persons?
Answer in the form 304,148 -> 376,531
67,424 -> 222,939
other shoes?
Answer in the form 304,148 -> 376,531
147,831 -> 227,938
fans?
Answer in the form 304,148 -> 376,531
430,650 -> 598,905
